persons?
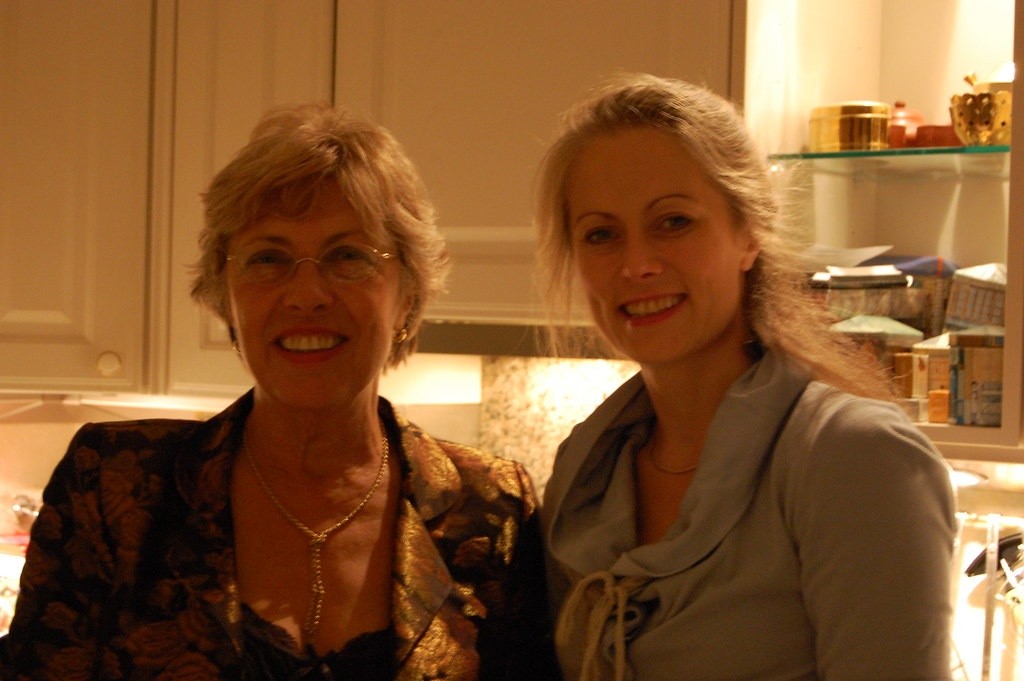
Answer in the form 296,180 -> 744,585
0,105 -> 542,680
542,73 -> 951,681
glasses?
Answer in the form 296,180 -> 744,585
218,242 -> 400,289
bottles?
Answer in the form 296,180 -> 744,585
888,102 -> 906,145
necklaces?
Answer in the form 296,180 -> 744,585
242,431 -> 388,635
649,437 -> 706,475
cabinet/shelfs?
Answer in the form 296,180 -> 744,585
0,0 -> 747,414
768,0 -> 1024,462
